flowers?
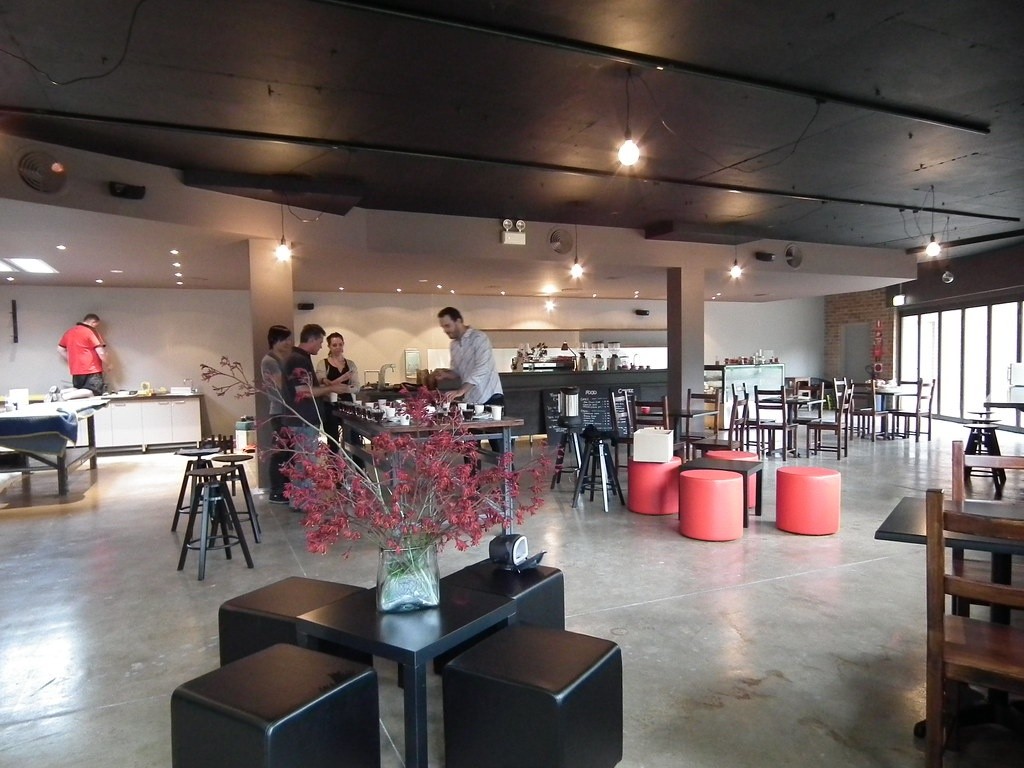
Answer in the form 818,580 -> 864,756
199,354 -> 564,559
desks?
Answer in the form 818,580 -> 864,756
854,391 -> 917,441
984,387 -> 1024,413
297,582 -> 518,768
637,410 -> 718,462
331,408 -> 524,535
756,398 -> 827,458
875,497 -> 1024,738
0,399 -> 112,495
679,458 -> 764,528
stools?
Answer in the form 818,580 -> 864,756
627,449 -> 840,542
169,558 -> 625,768
962,411 -> 1007,497
172,448 -> 261,581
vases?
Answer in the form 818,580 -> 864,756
375,534 -> 440,612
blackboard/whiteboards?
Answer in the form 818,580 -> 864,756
541,385 -> 642,446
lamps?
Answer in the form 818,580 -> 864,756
501,219 -> 526,245
561,339 -> 579,372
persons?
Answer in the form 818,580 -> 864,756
43,314 -> 113,404
437,307 -> 519,501
261,322 -> 361,504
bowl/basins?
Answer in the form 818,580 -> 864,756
642,407 -> 651,414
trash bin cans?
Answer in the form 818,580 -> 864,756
235,421 -> 257,454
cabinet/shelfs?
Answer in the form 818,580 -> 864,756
67,397 -> 202,453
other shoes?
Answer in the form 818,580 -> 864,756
269,489 -> 289,503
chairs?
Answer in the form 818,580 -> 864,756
609,375 -> 936,474
944,441 -> 1024,714
924,489 -> 1024,768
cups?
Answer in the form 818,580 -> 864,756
328,392 -> 503,425
5,395 -> 16,412
